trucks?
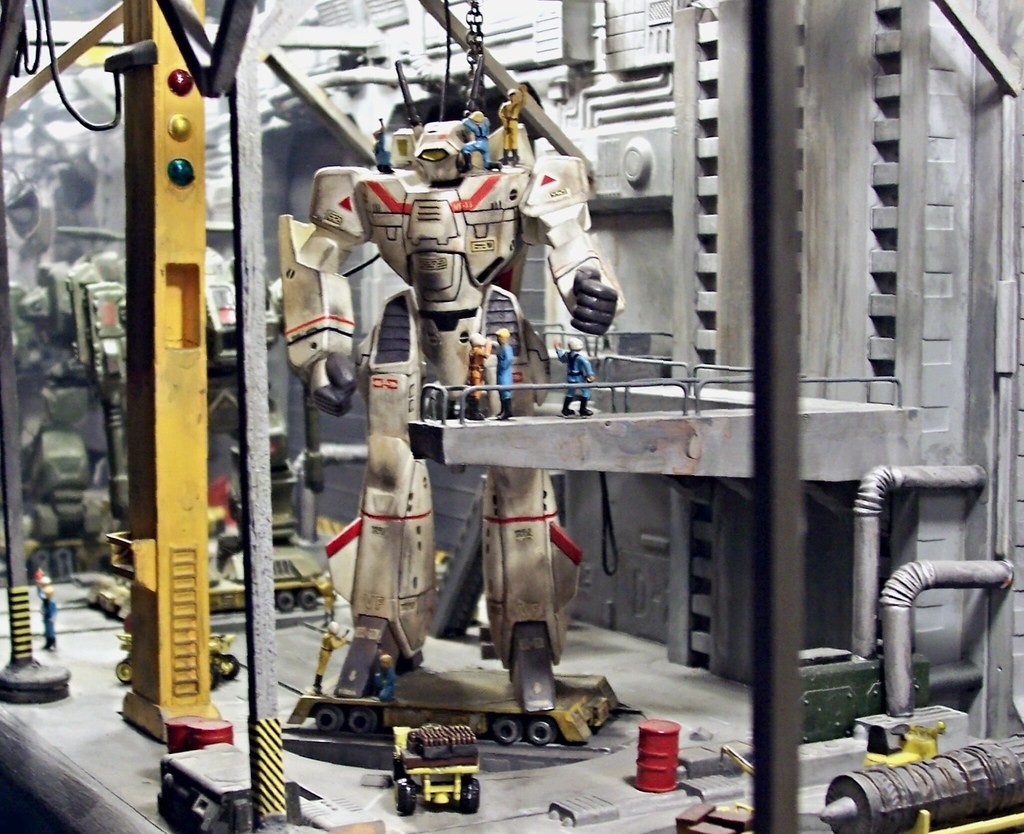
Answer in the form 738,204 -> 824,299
392,722 -> 482,816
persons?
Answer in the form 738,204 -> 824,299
278,120 -> 626,711
372,86 -> 526,175
311,570 -> 397,702
467,328 -> 514,421
36,583 -> 57,652
553,338 -> 594,416
